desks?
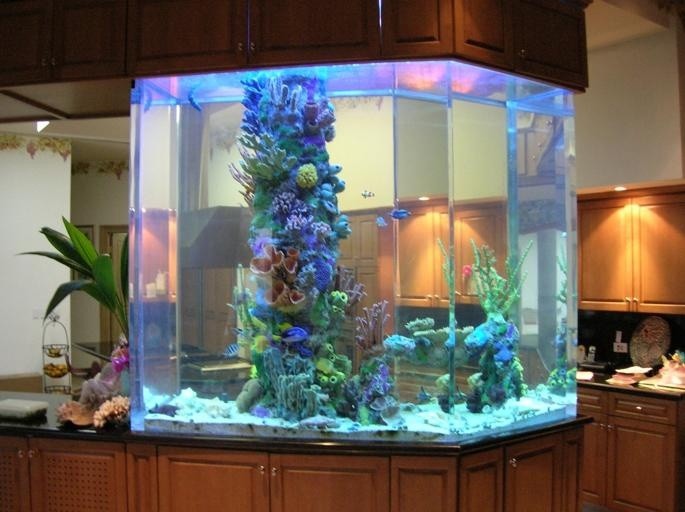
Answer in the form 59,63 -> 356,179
73,341 -> 251,376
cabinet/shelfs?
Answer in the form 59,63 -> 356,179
0,387 -> 685,511
577,178 -> 685,312
1,0 -> 591,123
180,195 -> 509,397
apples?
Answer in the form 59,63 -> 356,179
44,363 -> 67,376
